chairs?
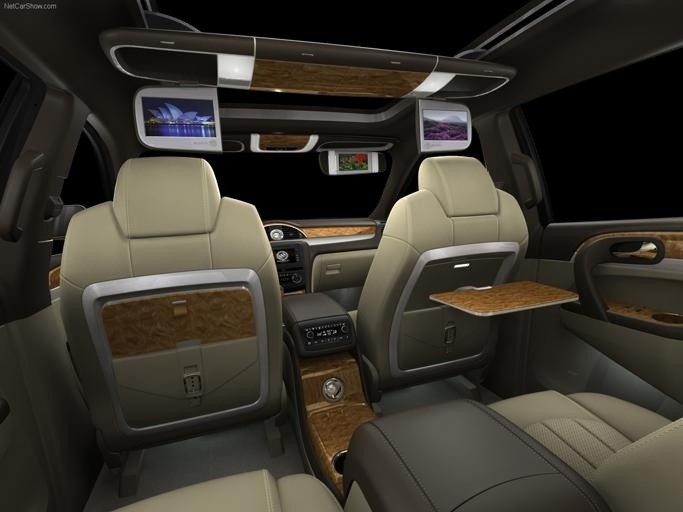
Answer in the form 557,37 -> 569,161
59,156 -> 284,451
111,467 -> 344,511
488,391 -> 683,511
347,155 -> 528,404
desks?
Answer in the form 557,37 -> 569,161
428,278 -> 581,319
